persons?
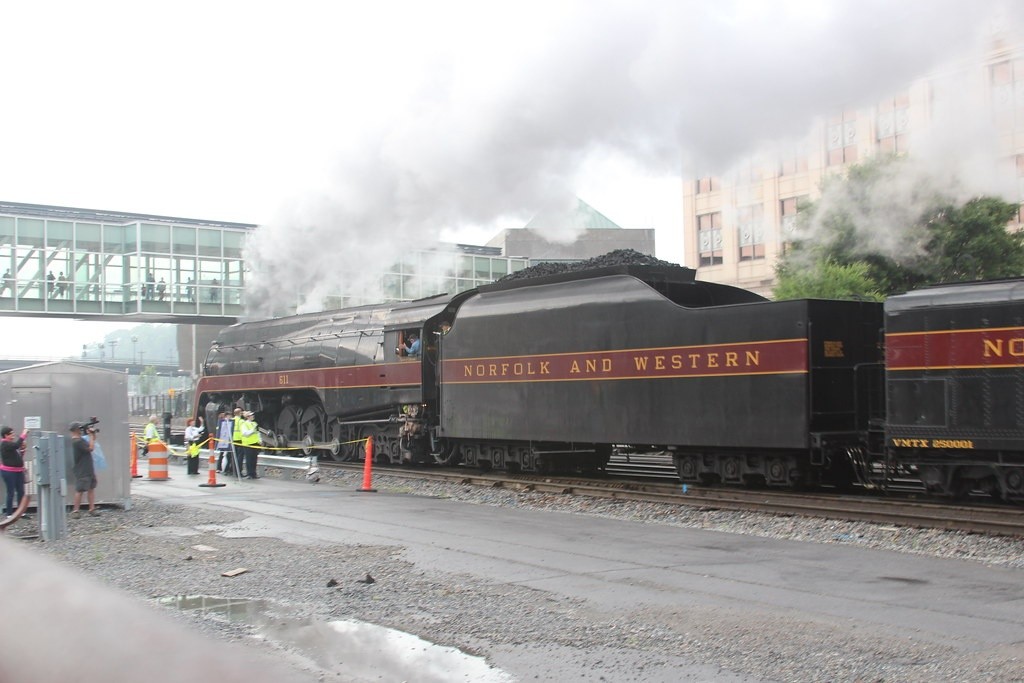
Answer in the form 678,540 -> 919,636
399,334 -> 420,355
47,271 -> 56,299
156,277 -> 166,300
2,269 -> 13,288
145,273 -> 155,300
215,407 -> 262,479
57,272 -> 67,296
186,277 -> 194,302
0,427 -> 31,519
67,421 -> 102,519
142,416 -> 160,455
208,278 -> 218,302
439,321 -> 451,334
185,416 -> 205,474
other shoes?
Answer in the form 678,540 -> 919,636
89,510 -> 100,516
20,513 -> 27,517
188,472 -> 200,474
72,512 -> 81,519
216,469 -> 220,472
248,473 -> 261,479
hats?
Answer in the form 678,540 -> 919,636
244,411 -> 254,417
69,421 -> 83,430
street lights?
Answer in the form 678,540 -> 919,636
130,335 -> 138,364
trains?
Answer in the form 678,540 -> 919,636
191,268 -> 1023,508
